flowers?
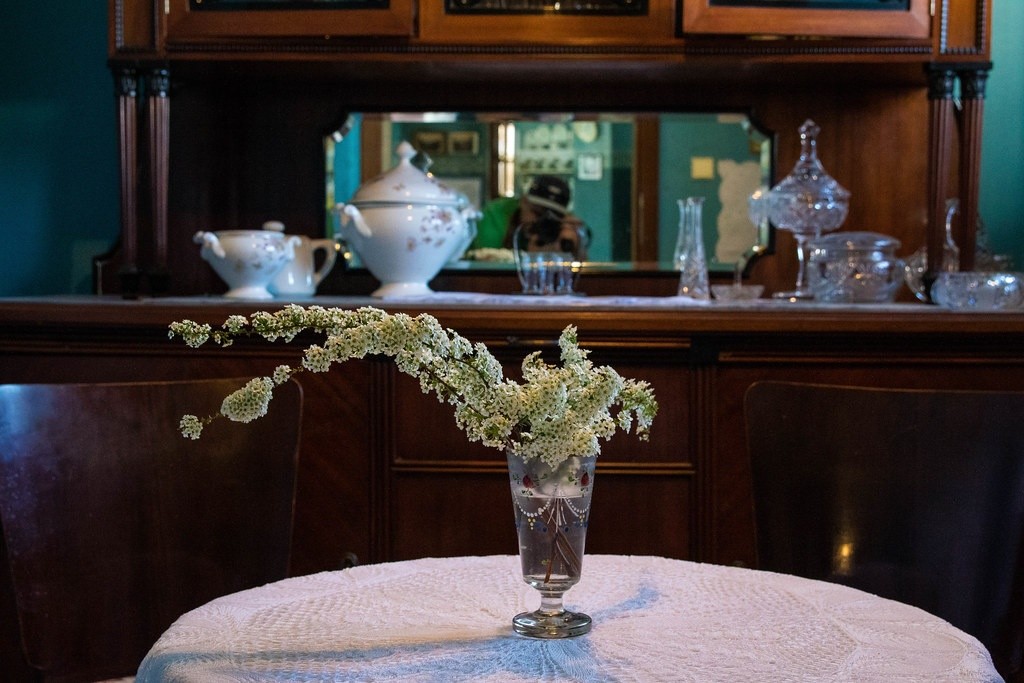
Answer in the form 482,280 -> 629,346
162,296 -> 661,468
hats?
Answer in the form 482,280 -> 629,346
524,173 -> 570,219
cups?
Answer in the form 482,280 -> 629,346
267,234 -> 338,298
808,231 -> 905,303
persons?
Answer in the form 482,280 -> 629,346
465,176 -> 587,261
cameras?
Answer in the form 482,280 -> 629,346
529,219 -> 563,247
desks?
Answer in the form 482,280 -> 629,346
134,553 -> 1005,683
0,292 -> 1023,683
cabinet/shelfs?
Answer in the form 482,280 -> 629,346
417,0 -> 991,65
107,0 -> 416,67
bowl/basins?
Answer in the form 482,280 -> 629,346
929,272 -> 1023,312
710,285 -> 765,300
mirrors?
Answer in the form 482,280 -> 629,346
324,107 -> 776,280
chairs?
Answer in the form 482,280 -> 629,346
1,371 -> 304,683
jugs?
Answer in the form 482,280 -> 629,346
330,142 -> 462,297
191,227 -> 301,298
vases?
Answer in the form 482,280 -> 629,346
497,445 -> 602,639
